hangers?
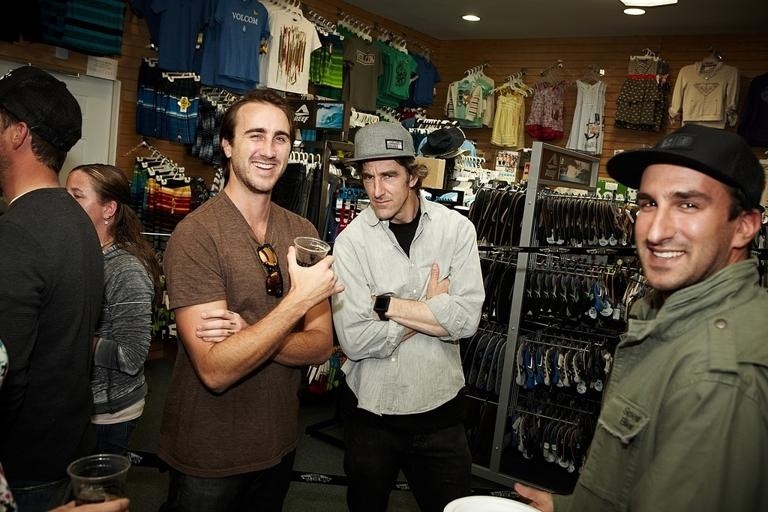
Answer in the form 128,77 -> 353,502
137,146 -> 195,189
410,115 -> 487,186
264,1 -> 435,58
286,149 -> 322,169
459,47 -> 724,97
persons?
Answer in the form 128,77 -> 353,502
1,342 -> 131,511
331,121 -> 486,511
514,125 -> 768,512
1,66 -> 102,511
65,163 -> 162,484
163,90 -> 346,512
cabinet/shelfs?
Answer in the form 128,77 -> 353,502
436,140 -> 646,505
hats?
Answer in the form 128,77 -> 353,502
345,121 -> 415,163
0,66 -> 81,151
420,126 -> 465,156
606,125 -> 765,213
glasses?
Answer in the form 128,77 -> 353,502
257,244 -> 283,298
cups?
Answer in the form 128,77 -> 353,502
66,453 -> 131,506
294,236 -> 331,267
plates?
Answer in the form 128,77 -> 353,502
444,495 -> 541,512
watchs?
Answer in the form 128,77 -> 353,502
374,295 -> 390,321
